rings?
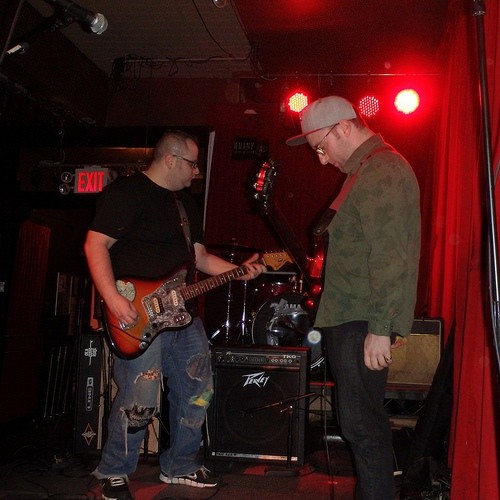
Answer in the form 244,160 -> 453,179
385,355 -> 392,361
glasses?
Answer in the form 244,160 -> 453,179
312,123 -> 340,156
173,155 -> 198,170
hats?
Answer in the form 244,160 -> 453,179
285,96 -> 358,146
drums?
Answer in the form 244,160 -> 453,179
246,281 -> 297,321
241,284 -> 330,373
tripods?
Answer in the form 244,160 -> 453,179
206,252 -> 253,349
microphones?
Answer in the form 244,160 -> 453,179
53,0 -> 108,36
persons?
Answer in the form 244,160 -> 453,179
286,96 -> 418,499
84,130 -> 267,500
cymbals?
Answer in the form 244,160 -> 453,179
201,242 -> 263,256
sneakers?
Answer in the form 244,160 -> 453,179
98,475 -> 134,500
158,466 -> 218,488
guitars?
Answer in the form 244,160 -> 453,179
252,159 -> 326,320
97,249 -> 294,359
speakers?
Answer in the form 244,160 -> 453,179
384,318 -> 444,389
73,333 -> 161,457
210,348 -> 309,465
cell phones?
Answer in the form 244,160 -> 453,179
358,348 -> 387,370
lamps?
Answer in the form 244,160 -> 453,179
58,167 -> 116,195
51,0 -> 109,37
